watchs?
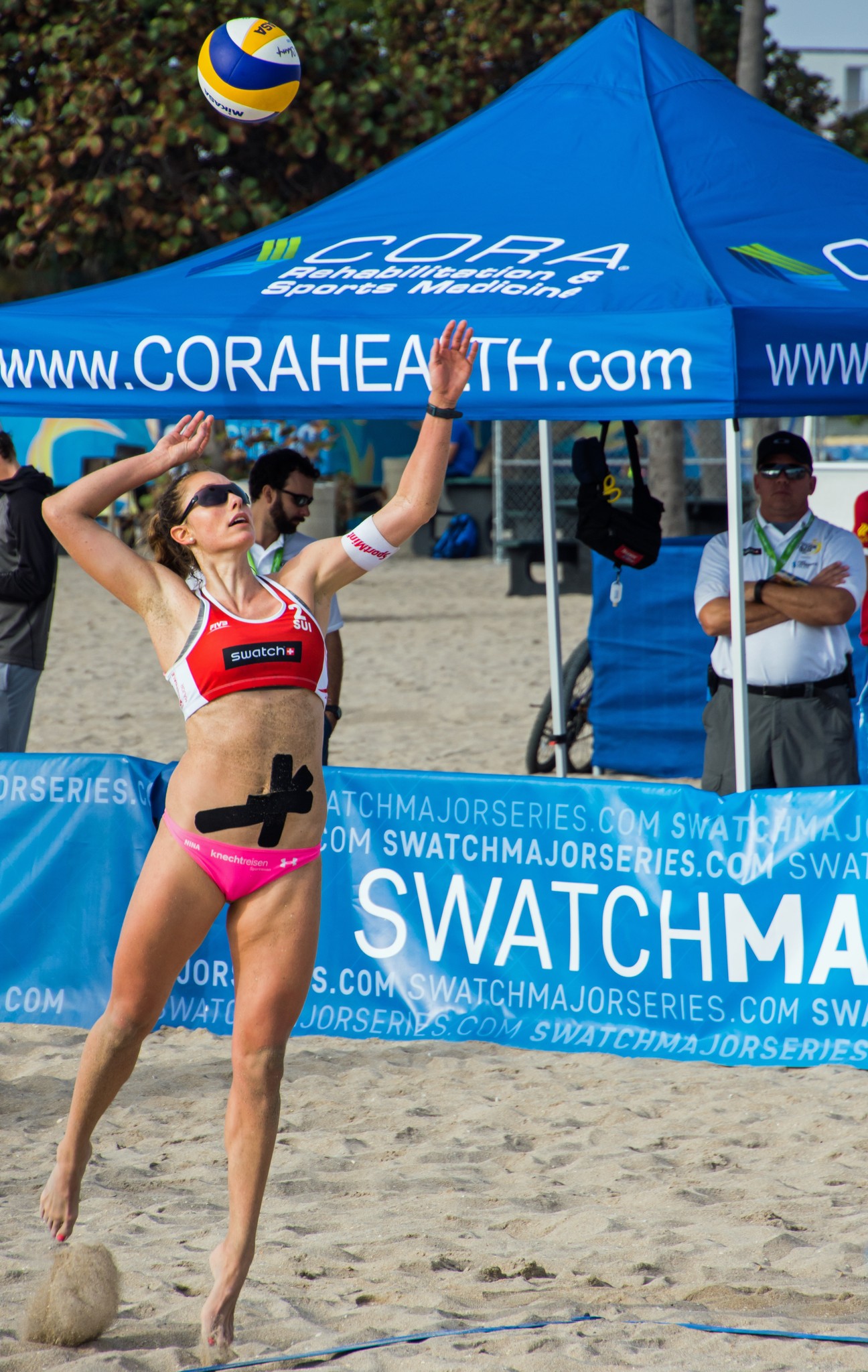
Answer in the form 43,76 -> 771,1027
325,705 -> 342,720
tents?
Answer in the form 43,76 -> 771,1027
0,10 -> 868,1069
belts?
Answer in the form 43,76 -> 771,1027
720,672 -> 850,699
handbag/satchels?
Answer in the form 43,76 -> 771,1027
574,418 -> 666,572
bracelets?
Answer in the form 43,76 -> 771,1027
754,579 -> 768,604
426,402 -> 462,419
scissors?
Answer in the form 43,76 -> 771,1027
603,476 -> 622,504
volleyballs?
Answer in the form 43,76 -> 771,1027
197,16 -> 301,124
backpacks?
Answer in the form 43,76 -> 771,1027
432,513 -> 482,559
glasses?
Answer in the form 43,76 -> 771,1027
758,463 -> 810,480
179,482 -> 251,524
282,489 -> 315,508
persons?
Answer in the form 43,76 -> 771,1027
693,430 -> 867,796
443,421 -> 477,479
39,317 -> 481,1363
0,429 -> 61,755
240,448 -> 344,766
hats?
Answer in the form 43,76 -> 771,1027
757,431 -> 813,466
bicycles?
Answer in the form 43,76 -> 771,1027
524,637 -> 595,775
